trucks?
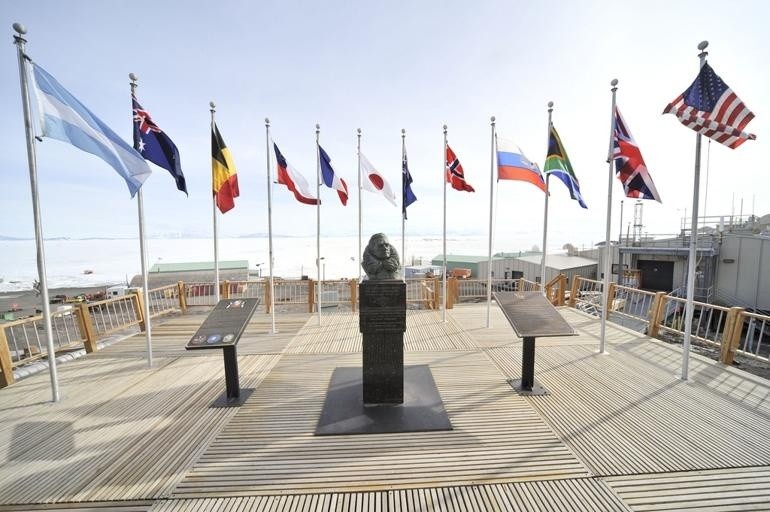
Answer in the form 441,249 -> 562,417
106,285 -> 142,299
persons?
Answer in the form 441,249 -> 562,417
361,232 -> 402,280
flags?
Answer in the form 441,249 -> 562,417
544,126 -> 589,210
358,151 -> 398,210
494,135 -> 550,197
606,105 -> 663,204
270,140 -> 321,206
320,149 -> 349,206
446,143 -> 476,193
401,152 -> 418,220
209,121 -> 240,213
130,96 -> 189,199
24,59 -> 153,199
662,61 -> 757,150
